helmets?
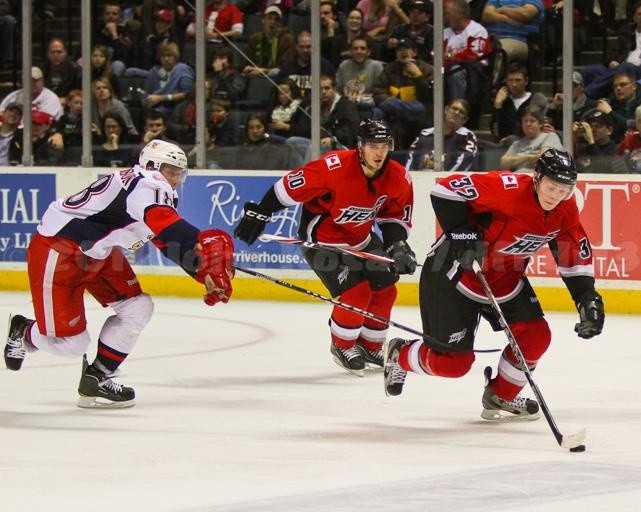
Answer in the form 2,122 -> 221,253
534,147 -> 578,185
356,117 -> 395,153
139,139 -> 189,173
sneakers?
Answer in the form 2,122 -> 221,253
330,340 -> 365,371
3,314 -> 35,371
482,368 -> 540,415
355,342 -> 385,368
384,337 -> 406,397
79,351 -> 135,403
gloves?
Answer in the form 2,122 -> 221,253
442,217 -> 483,272
574,288 -> 605,338
382,235 -> 418,275
194,228 -> 234,276
233,202 -> 273,247
194,268 -> 233,306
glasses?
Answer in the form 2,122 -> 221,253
33,76 -> 45,83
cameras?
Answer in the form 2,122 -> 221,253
577,126 -> 585,133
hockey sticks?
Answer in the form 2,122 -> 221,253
473,261 -> 585,450
233,264 -> 501,353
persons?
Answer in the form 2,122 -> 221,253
234,115 -> 416,372
382,147 -> 605,419
2,136 -> 238,404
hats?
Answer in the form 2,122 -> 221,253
409,0 -> 427,12
397,38 -> 416,50
571,70 -> 583,85
3,103 -> 24,117
586,110 -> 614,127
31,110 -> 50,125
